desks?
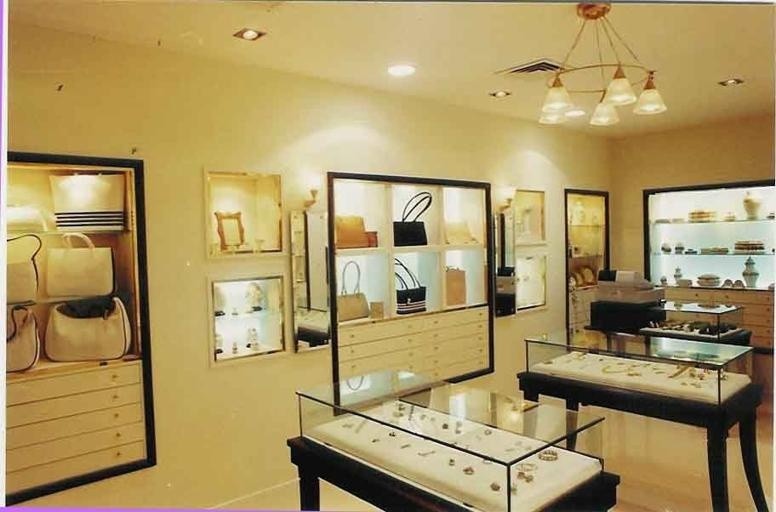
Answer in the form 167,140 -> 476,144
516,370 -> 768,511
585,326 -> 752,366
287,437 -> 620,511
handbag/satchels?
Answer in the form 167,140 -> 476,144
45,247 -> 118,296
396,286 -> 426,314
44,296 -> 131,363
336,293 -> 370,321
7,260 -> 38,305
394,221 -> 427,246
335,215 -> 369,247
446,270 -> 466,305
49,173 -> 127,230
6,312 -> 40,373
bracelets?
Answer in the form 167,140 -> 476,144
539,450 -> 559,461
514,461 -> 537,471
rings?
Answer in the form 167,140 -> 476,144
463,466 -> 474,474
449,458 -> 456,465
484,429 -> 491,434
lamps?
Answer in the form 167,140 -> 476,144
500,185 -> 513,211
538,2 -> 665,127
303,177 -> 323,209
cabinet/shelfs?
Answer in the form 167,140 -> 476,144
566,187 -> 612,352
206,273 -> 287,368
328,172 -> 494,415
642,179 -> 775,356
7,151 -> 159,506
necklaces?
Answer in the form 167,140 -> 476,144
602,362 -> 628,373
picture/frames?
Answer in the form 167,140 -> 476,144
215,212 -> 245,250
